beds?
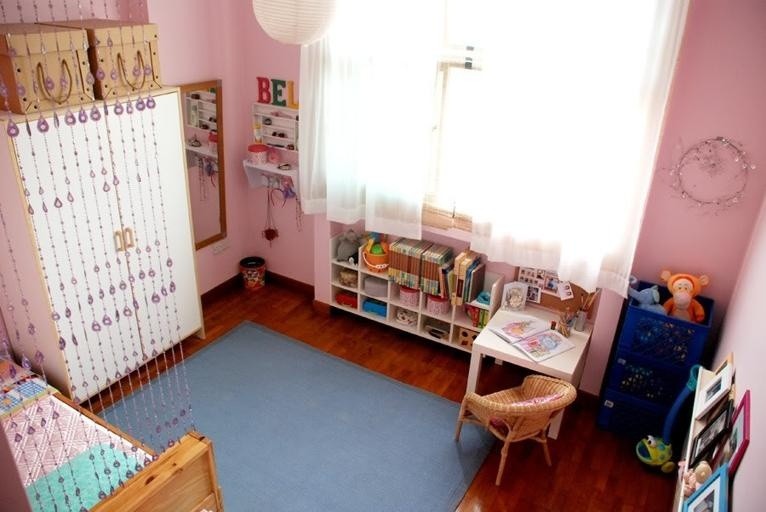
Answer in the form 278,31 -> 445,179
0,352 -> 224,512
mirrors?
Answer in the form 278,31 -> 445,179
177,79 -> 227,251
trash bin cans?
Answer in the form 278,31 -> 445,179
239,256 -> 266,289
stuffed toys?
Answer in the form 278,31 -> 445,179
363,232 -> 389,273
683,460 -> 712,499
336,228 -> 359,262
659,270 -> 709,323
628,274 -> 666,353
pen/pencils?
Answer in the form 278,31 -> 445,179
581,289 -> 601,312
559,306 -> 580,338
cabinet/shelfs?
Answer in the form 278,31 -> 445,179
0,88 -> 206,405
328,231 -> 506,359
250,102 -> 299,154
184,88 -> 217,133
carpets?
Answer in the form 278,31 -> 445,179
96,319 -> 498,512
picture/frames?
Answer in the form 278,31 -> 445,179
500,280 -> 529,312
681,351 -> 750,512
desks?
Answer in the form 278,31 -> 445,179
465,305 -> 594,440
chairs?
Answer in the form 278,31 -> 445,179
455,374 -> 578,486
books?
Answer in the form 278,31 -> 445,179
487,316 -> 575,363
388,237 -> 485,306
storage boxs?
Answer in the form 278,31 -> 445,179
597,279 -> 715,447
34,18 -> 162,100
0,24 -> 96,114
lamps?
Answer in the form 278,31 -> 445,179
252,0 -> 346,45
